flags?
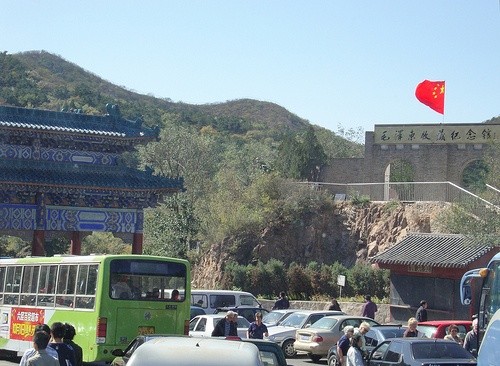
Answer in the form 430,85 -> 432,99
415,80 -> 445,115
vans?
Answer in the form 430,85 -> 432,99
156,287 -> 265,309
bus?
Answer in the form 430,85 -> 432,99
459,253 -> 500,354
0,253 -> 190,365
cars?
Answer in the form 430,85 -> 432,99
366,338 -> 478,366
266,311 -> 347,359
188,307 -> 206,318
256,309 -> 298,330
365,326 -> 429,356
292,317 -> 382,361
114,334 -> 291,366
415,320 -> 474,341
187,315 -> 253,339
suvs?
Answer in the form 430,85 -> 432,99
212,306 -> 269,323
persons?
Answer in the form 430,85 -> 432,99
444,324 -> 463,347
362,295 -> 378,320
337,321 -> 371,366
211,311 -> 238,337
463,318 -> 478,359
271,291 -> 290,310
329,300 -> 341,311
404,317 -> 426,337
171,290 -> 180,301
416,300 -> 427,322
19,322 -> 83,366
246,312 -> 268,339
151,288 -> 160,298
